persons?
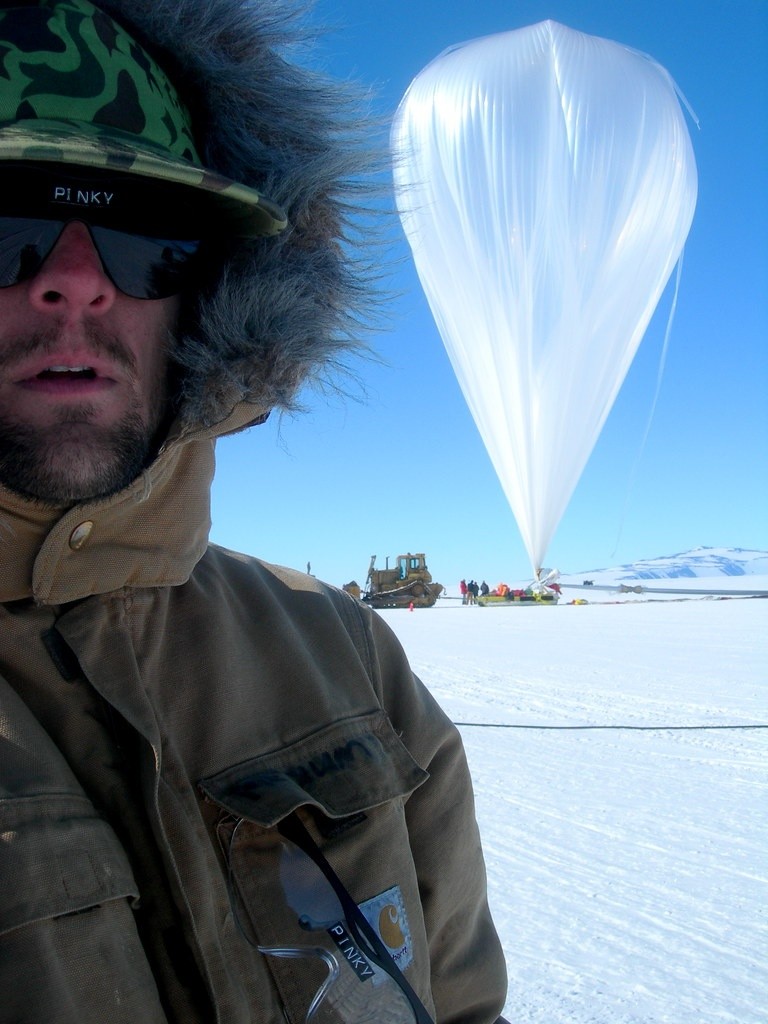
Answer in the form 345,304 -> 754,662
0,0 -> 507,1024
460,578 -> 509,605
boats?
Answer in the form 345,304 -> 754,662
476,592 -> 559,606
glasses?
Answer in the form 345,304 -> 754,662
0,158 -> 237,301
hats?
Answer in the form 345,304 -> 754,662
1,0 -> 291,240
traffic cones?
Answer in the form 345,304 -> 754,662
409,601 -> 413,611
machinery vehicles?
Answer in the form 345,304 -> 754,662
343,552 -> 443,608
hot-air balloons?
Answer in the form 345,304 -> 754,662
388,18 -> 701,595
